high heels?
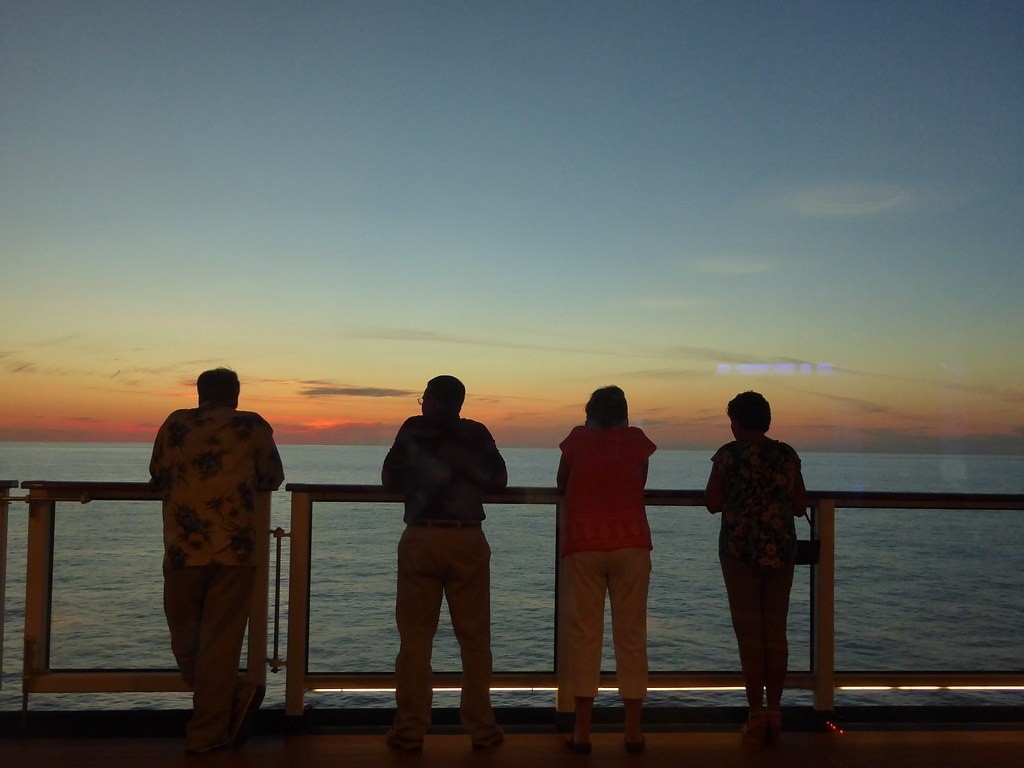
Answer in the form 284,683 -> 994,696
742,711 -> 767,746
768,711 -> 781,745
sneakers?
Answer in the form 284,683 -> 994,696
472,728 -> 505,750
384,729 -> 424,752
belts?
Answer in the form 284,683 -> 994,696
410,521 -> 482,529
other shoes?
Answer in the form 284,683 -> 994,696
183,734 -> 230,753
230,683 -> 266,752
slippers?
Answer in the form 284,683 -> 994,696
566,733 -> 592,754
624,731 -> 646,753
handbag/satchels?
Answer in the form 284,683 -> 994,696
795,512 -> 821,565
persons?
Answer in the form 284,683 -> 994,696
150,369 -> 283,754
382,375 -> 507,751
704,391 -> 808,743
556,386 -> 657,755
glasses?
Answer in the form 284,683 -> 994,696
417,398 -> 424,405
